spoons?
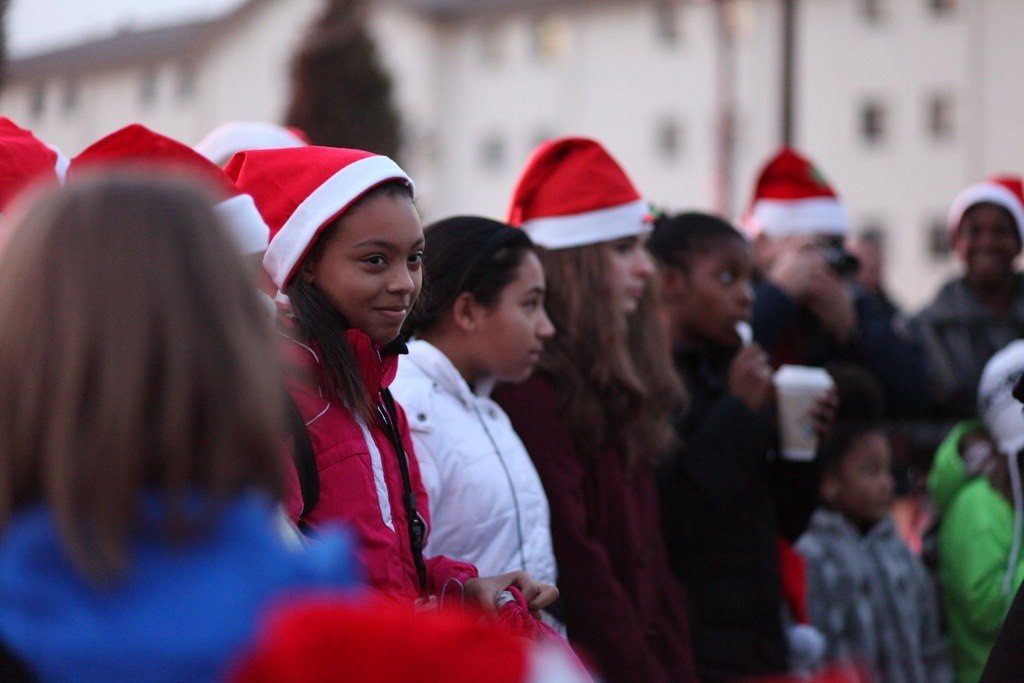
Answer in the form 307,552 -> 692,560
735,320 -> 753,348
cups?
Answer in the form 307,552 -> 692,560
771,364 -> 834,459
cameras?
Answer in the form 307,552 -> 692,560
816,241 -> 859,278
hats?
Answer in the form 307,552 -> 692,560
222,146 -> 415,294
739,142 -> 849,238
0,117 -> 69,250
945,173 -> 1024,241
506,135 -> 654,250
189,120 -> 312,175
63,124 -> 270,259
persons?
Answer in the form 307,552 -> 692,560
0,120 -> 1024,683
